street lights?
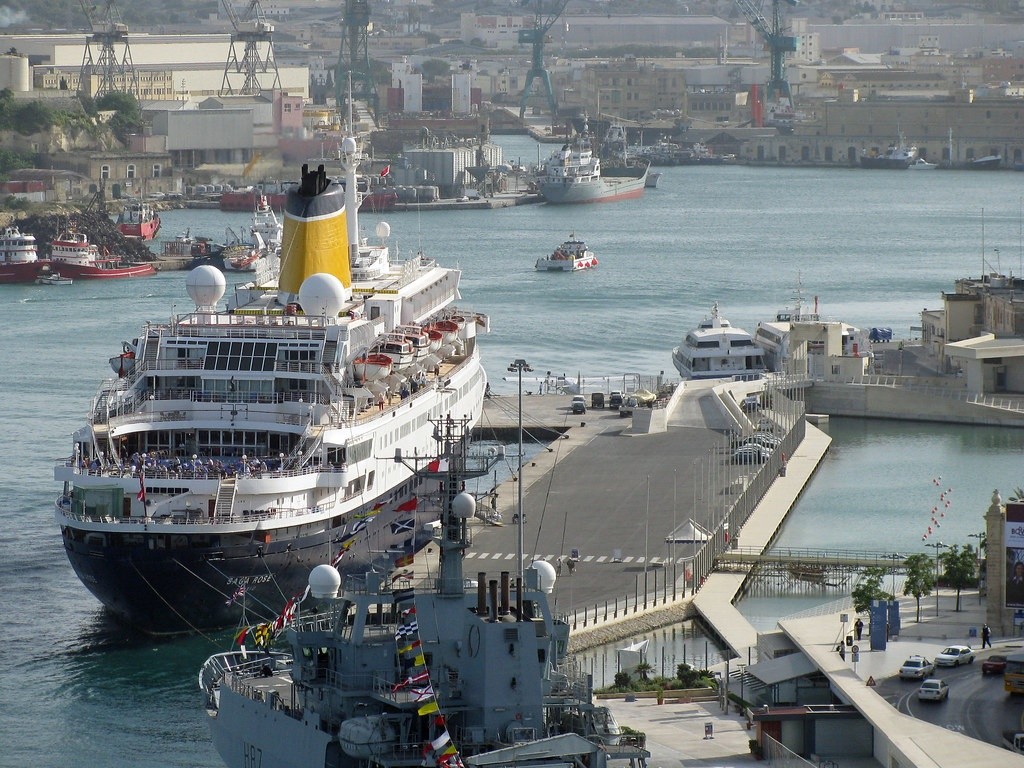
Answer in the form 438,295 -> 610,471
967,532 -> 986,605
508,359 -> 533,586
880,554 -> 908,599
926,541 -> 948,616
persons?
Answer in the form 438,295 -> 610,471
378,374 -> 427,411
490,494 -> 499,511
840,641 -> 845,661
1007,562 -> 1024,604
982,623 -> 991,649
567,558 -> 574,576
555,558 -> 561,576
855,618 -> 864,640
83,453 -> 268,479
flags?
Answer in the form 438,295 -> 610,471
428,457 -> 448,472
233,498 -> 464,768
137,477 -> 145,503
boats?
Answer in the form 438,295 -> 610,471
672,270 -> 873,381
36,263 -> 73,285
530,89 -> 736,204
859,115 -> 1002,171
534,230 -> 594,271
0,224 -> 54,284
52,66 -> 650,768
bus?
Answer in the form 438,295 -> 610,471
1004,646 -> 1024,692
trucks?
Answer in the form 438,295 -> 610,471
592,391 -> 638,415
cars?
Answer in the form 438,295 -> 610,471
899,645 -> 975,702
982,655 -> 1007,675
733,433 -> 781,464
146,191 -> 182,201
571,394 -> 585,414
456,196 -> 469,202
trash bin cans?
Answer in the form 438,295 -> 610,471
969,627 -> 977,637
779,467 -> 786,477
732,538 -> 739,549
581,422 -> 585,427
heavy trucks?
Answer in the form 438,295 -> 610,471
191,240 -> 218,258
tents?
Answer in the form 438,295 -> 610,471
665,518 -> 712,576
